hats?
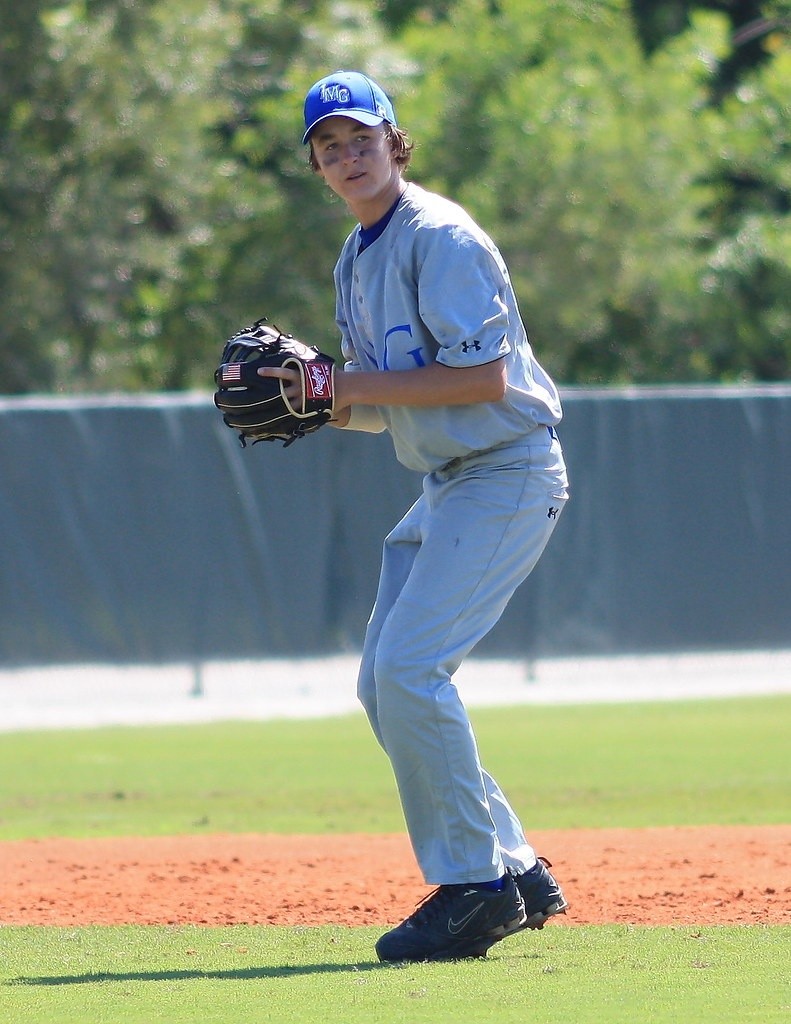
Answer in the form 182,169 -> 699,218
302,70 -> 395,145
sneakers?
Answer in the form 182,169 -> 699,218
375,874 -> 526,964
512,857 -> 568,930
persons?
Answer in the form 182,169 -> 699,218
213,70 -> 569,963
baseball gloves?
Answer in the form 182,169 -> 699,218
211,315 -> 337,450
